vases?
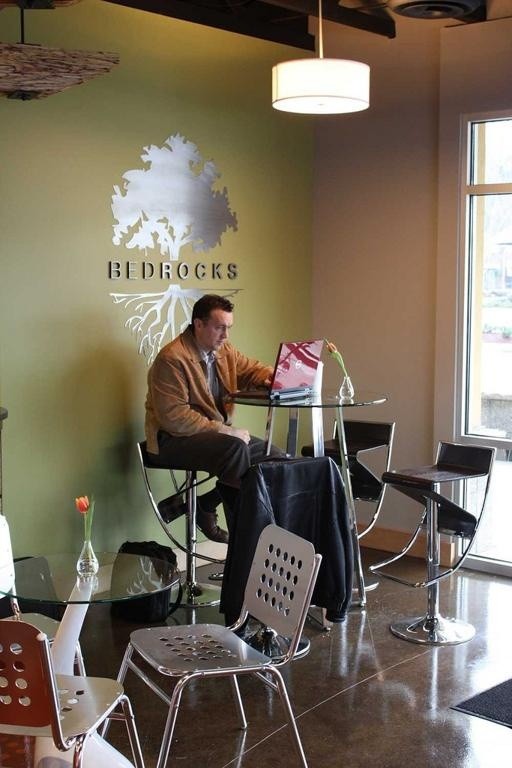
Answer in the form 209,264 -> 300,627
338,375 -> 355,398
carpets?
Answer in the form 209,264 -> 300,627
448,677 -> 512,727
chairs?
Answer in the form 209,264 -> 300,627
136,440 -> 225,610
2,620 -> 145,768
301,419 -> 398,593
99,524 -> 322,767
1,523 -> 87,678
382,440 -> 495,644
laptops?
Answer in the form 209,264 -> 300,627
228,338 -> 324,401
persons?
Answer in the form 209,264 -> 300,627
145,294 -> 286,543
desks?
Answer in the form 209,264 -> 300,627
1,551 -> 181,768
223,389 -> 387,608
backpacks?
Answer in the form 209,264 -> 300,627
110,540 -> 183,623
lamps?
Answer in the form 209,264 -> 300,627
271,1 -> 373,115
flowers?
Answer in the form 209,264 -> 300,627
323,337 -> 351,377
74,493 -> 96,541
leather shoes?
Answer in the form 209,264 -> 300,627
196,496 -> 228,543
